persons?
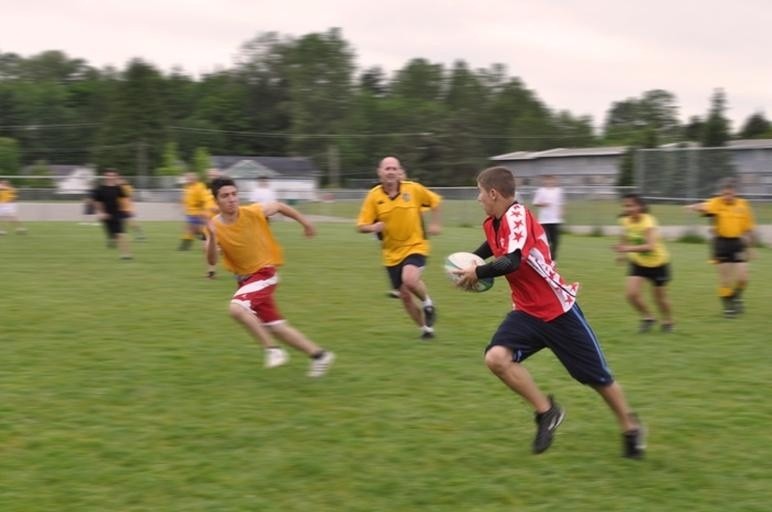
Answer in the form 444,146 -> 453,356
0,179 -> 27,234
84,167 -> 138,263
177,169 -> 339,382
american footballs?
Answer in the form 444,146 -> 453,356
444,252 -> 493,291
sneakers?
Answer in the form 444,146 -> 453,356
621,414 -> 649,458
386,289 -> 403,298
639,316 -> 654,333
419,303 -> 436,339
721,297 -> 744,317
660,318 -> 674,331
307,350 -> 336,379
532,393 -> 566,454
264,343 -> 289,369
